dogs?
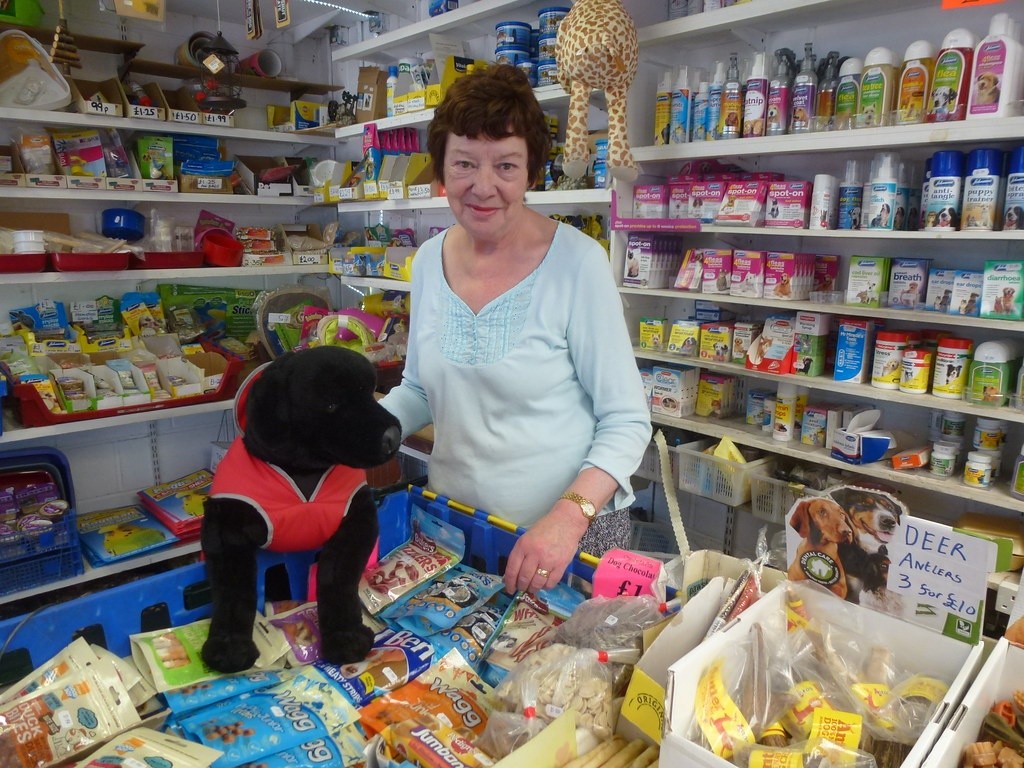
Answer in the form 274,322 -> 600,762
788,487 -> 903,612
199,346 -> 402,674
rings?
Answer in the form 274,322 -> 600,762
536,567 -> 551,578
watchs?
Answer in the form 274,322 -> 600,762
559,492 -> 597,527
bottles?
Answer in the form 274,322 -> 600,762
654,20 -> 1024,502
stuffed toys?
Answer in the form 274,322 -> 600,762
199,345 -> 402,674
555,0 -> 640,181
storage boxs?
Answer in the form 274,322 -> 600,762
621,157 -> 1023,473
0,55 -> 488,429
0,429 -> 1024,768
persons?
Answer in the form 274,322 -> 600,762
379,70 -> 654,594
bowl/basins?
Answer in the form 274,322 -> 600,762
204,232 -> 243,264
198,228 -> 235,249
102,209 -> 144,240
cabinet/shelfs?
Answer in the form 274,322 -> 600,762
621,0 -> 1024,624
0,0 -> 329,611
330,0 -> 619,461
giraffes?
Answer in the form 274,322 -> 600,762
554,0 -> 639,181
